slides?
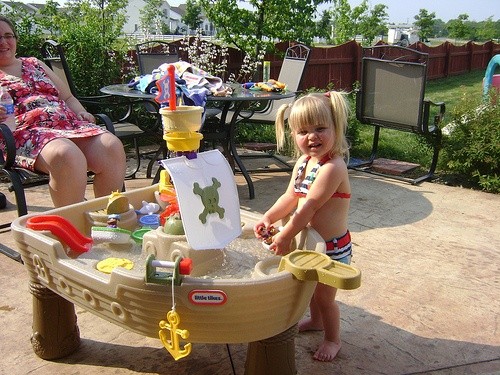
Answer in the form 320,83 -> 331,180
25,214 -> 93,253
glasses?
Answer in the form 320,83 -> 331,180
0,35 -> 16,41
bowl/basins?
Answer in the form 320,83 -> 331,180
139,214 -> 160,230
132,229 -> 151,245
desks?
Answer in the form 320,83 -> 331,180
100,84 -> 296,200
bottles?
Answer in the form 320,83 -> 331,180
263,61 -> 270,82
0,87 -> 16,133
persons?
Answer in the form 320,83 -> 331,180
0,16 -> 126,208
253,91 -> 353,361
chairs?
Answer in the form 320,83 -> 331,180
347,45 -> 446,185
214,44 -> 311,173
0,40 -> 220,264
